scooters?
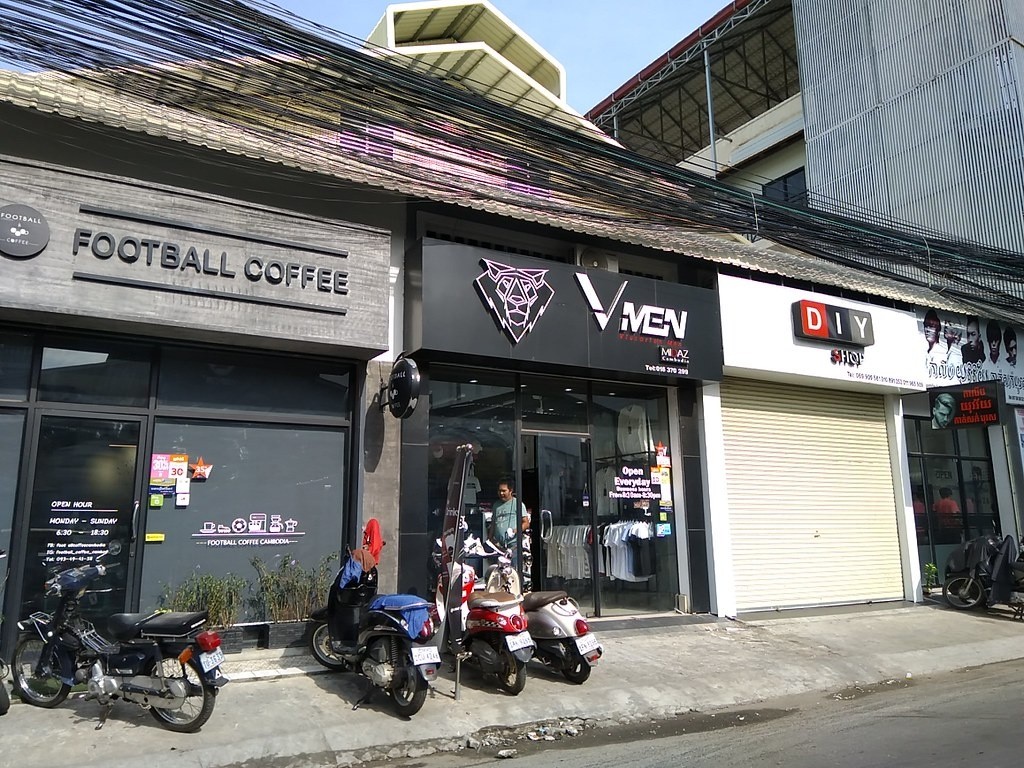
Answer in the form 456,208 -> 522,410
422,537 -> 539,695
482,539 -> 603,684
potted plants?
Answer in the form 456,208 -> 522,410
249,551 -> 326,649
921,562 -> 937,595
156,566 -> 245,654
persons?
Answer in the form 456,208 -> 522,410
961,316 -> 986,369
933,487 -> 963,544
489,477 -> 533,595
943,314 -> 963,367
931,393 -> 957,429
913,491 -> 928,545
980,320 -> 1015,376
1003,327 -> 1024,377
923,308 -> 947,368
971,466 -> 994,513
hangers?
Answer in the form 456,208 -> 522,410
600,454 -> 649,471
550,512 -> 653,527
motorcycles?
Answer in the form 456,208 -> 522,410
307,537 -> 442,716
0,539 -> 230,733
942,520 -> 1024,610
